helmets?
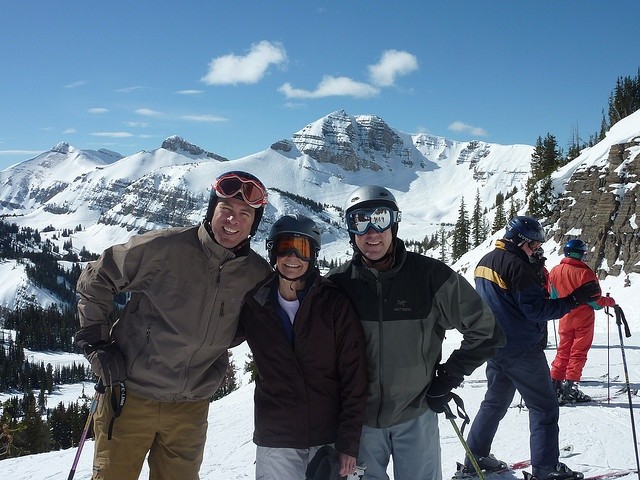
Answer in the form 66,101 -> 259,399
344,184 -> 400,228
504,216 -> 545,252
207,171 -> 268,236
265,215 -> 321,253
563,240 -> 588,261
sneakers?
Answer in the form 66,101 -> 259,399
533,465 -> 583,479
463,453 -> 506,472
561,380 -> 591,402
547,377 -> 561,396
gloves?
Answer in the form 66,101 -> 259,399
426,376 -> 453,415
74,324 -> 126,387
571,280 -> 602,309
604,296 -> 615,307
305,446 -> 367,480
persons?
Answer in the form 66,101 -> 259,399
74,171 -> 275,479
530,246 -> 549,350
462,215 -> 600,479
228,213 -> 368,479
323,184 -> 508,480
547,238 -> 615,406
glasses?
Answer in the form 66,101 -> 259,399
347,208 -> 401,234
519,233 -> 543,252
272,236 -> 315,261
209,176 -> 268,208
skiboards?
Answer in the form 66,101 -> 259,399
509,386 -> 638,411
451,446 -> 639,480
578,373 -> 618,382
455,380 -> 489,388
